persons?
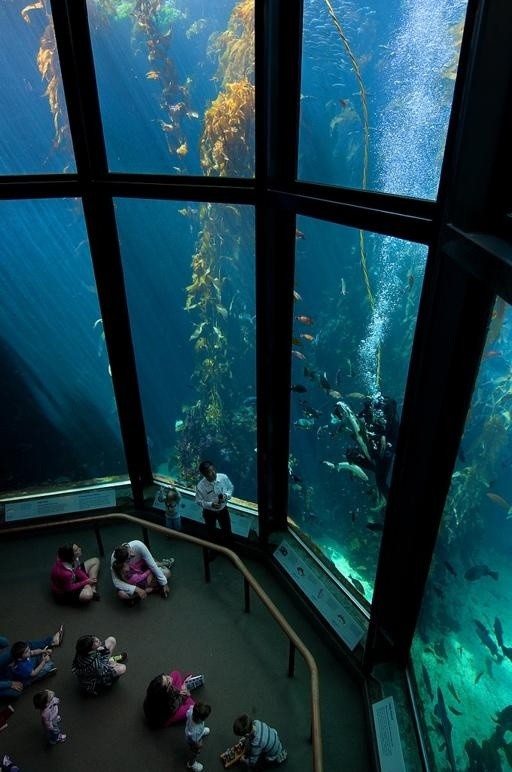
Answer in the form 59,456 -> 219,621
72,634 -> 128,697
233,713 -> 287,765
0,624 -> 67,731
346,396 -> 400,532
158,486 -> 182,537
143,670 -> 198,728
196,461 -> 235,563
50,542 -> 101,603
185,701 -> 211,772
33,688 -> 67,745
0,752 -> 20,772
109,540 -> 175,608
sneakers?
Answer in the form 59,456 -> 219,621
57,716 -> 61,722
50,734 -> 66,744
187,760 -> 203,772
204,727 -> 210,735
113,652 -> 127,662
162,557 -> 174,568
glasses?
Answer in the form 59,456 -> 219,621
166,675 -> 170,689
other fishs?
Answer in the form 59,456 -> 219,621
298,1 -> 467,138
289,214 -> 424,620
403,297 -> 512,772
240,396 -> 257,407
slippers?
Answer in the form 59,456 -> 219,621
55,625 -> 64,646
